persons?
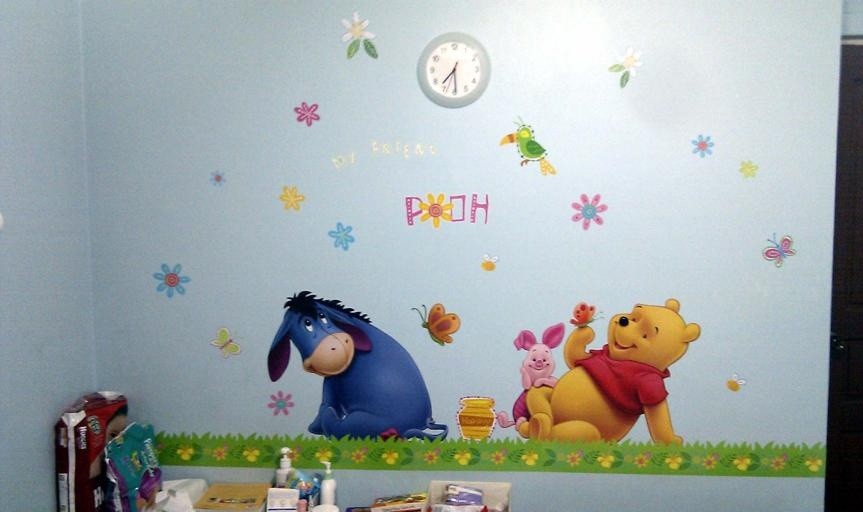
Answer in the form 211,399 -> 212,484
89,405 -> 128,479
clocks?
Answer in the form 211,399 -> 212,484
417,32 -> 492,108
